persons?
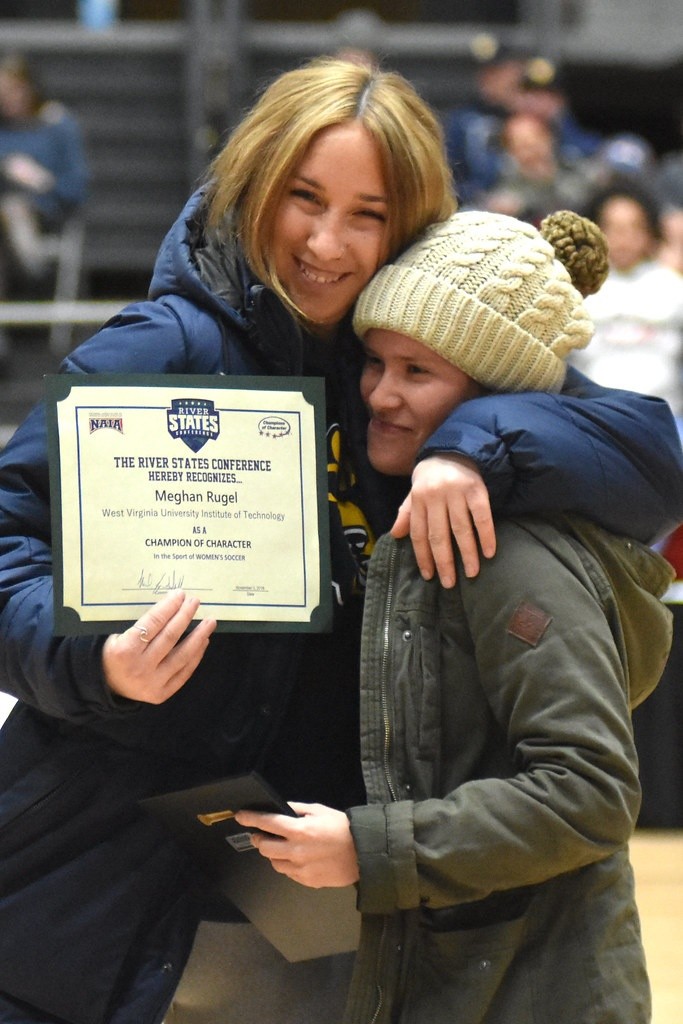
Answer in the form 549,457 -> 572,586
233,208 -> 677,1024
1,33 -> 682,1024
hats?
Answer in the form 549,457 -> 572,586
350,208 -> 610,395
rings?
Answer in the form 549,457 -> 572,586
132,625 -> 150,647
